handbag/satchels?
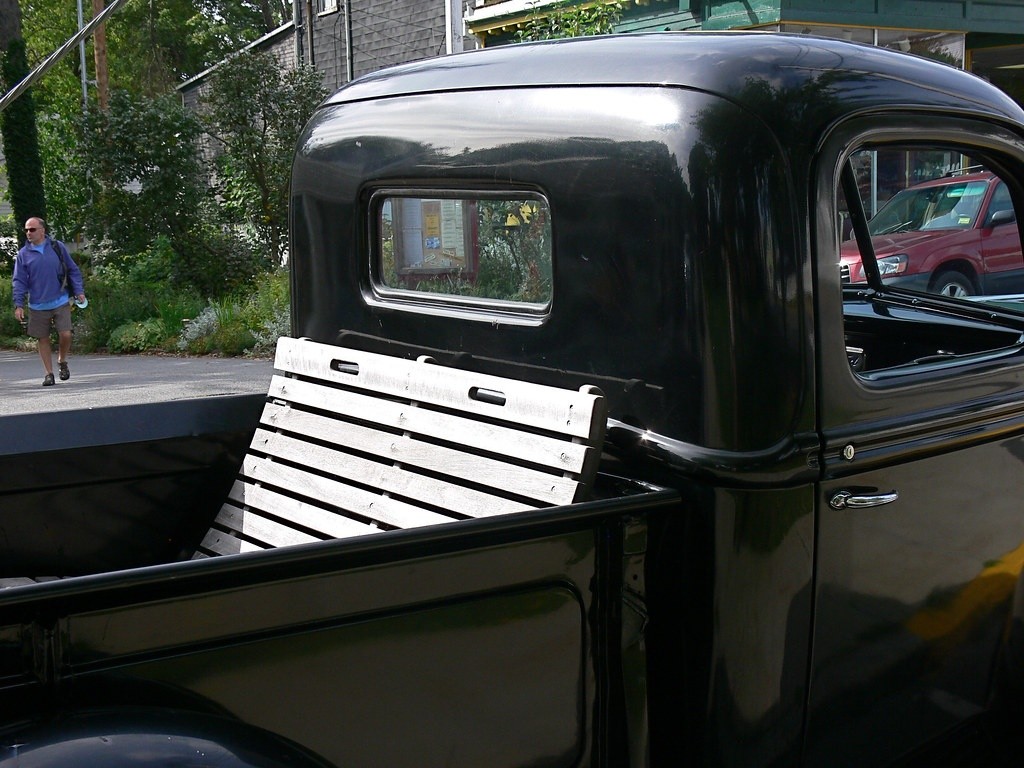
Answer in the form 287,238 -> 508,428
52,241 -> 72,292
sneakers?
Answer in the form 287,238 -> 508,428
57,361 -> 70,380
43,374 -> 55,385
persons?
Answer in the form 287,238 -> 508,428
12,218 -> 87,386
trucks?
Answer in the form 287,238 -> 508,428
1,28 -> 1023,768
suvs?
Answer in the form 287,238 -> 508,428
839,164 -> 1023,298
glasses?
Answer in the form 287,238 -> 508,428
23,228 -> 41,233
